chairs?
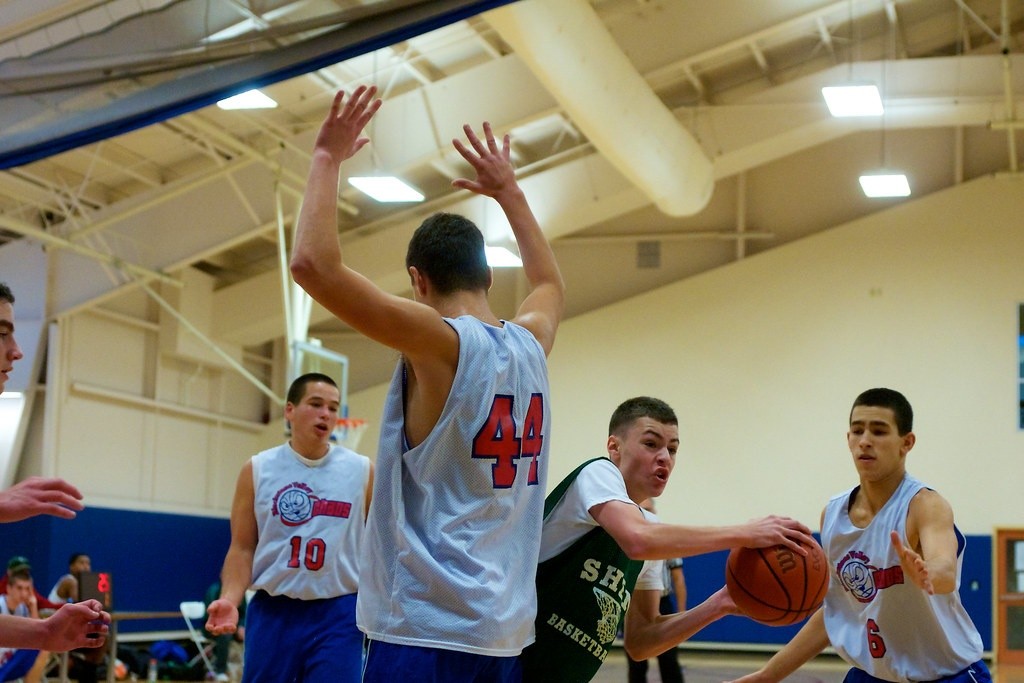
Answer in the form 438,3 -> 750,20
179,601 -> 219,679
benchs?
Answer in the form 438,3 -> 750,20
109,611 -> 203,644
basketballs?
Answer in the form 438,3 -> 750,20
725,525 -> 832,626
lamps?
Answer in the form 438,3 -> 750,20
858,0 -> 912,202
346,51 -> 428,204
821,0 -> 886,118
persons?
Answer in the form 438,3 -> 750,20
201,571 -> 247,682
621,496 -> 687,683
0,556 -> 67,610
722,386 -> 994,683
47,552 -> 91,604
0,564 -> 49,683
517,396 -> 816,683
205,373 -> 374,683
0,284 -> 110,651
289,85 -> 566,683
